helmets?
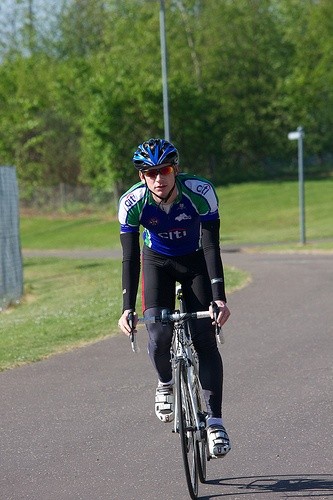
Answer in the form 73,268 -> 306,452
132,137 -> 179,172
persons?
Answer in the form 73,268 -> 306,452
118,138 -> 231,456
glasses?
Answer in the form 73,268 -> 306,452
141,166 -> 175,177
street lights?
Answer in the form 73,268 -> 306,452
288,130 -> 307,245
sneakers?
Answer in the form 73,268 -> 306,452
154,381 -> 175,424
205,418 -> 231,460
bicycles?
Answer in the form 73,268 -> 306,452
128,285 -> 224,500
